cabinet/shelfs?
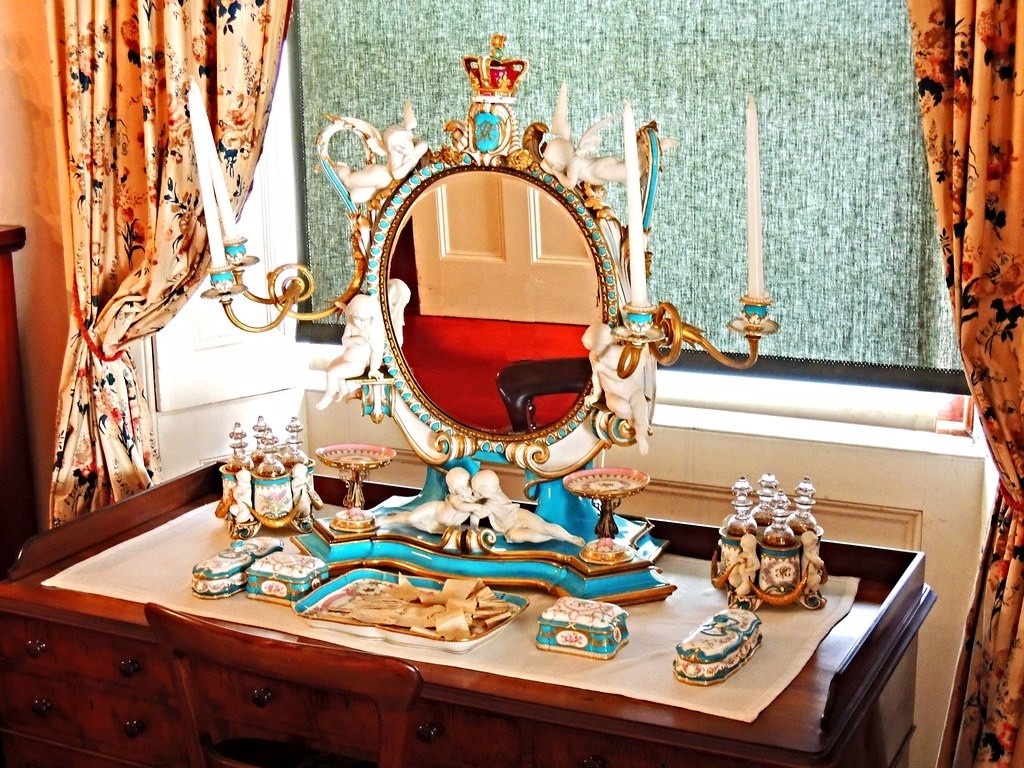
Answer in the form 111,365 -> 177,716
0,460 -> 938,768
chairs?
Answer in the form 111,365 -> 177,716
143,602 -> 424,767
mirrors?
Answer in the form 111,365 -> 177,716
202,33 -> 781,608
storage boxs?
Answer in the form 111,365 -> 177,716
193,536 -> 284,601
243,551 -> 330,607
536,596 -> 630,661
673,607 -> 765,688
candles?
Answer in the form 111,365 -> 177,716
187,75 -> 241,269
744,91 -> 765,299
618,98 -> 648,309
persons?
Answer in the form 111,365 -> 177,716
540,137 -> 678,190
801,531 -> 824,594
290,463 -> 310,518
316,294 -> 385,410
376,466 -> 586,548
729,534 -> 761,596
581,321 -> 653,456
335,124 -> 429,203
229,469 -> 254,523
388,278 -> 412,349
